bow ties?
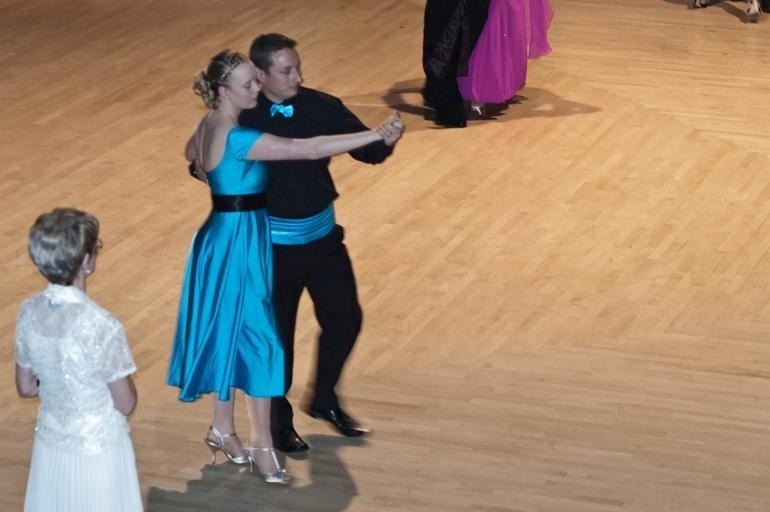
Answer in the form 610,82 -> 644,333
269,101 -> 295,119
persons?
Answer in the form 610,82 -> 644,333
164,49 -> 407,484
14,208 -> 144,512
189,33 -> 406,454
457,1 -> 553,115
421,2 -> 489,128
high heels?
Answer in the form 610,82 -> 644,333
246,446 -> 293,486
203,424 -> 251,467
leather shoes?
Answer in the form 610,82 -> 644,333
298,400 -> 365,438
423,82 -> 477,131
273,427 -> 309,453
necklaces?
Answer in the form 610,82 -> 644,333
219,108 -> 238,124
